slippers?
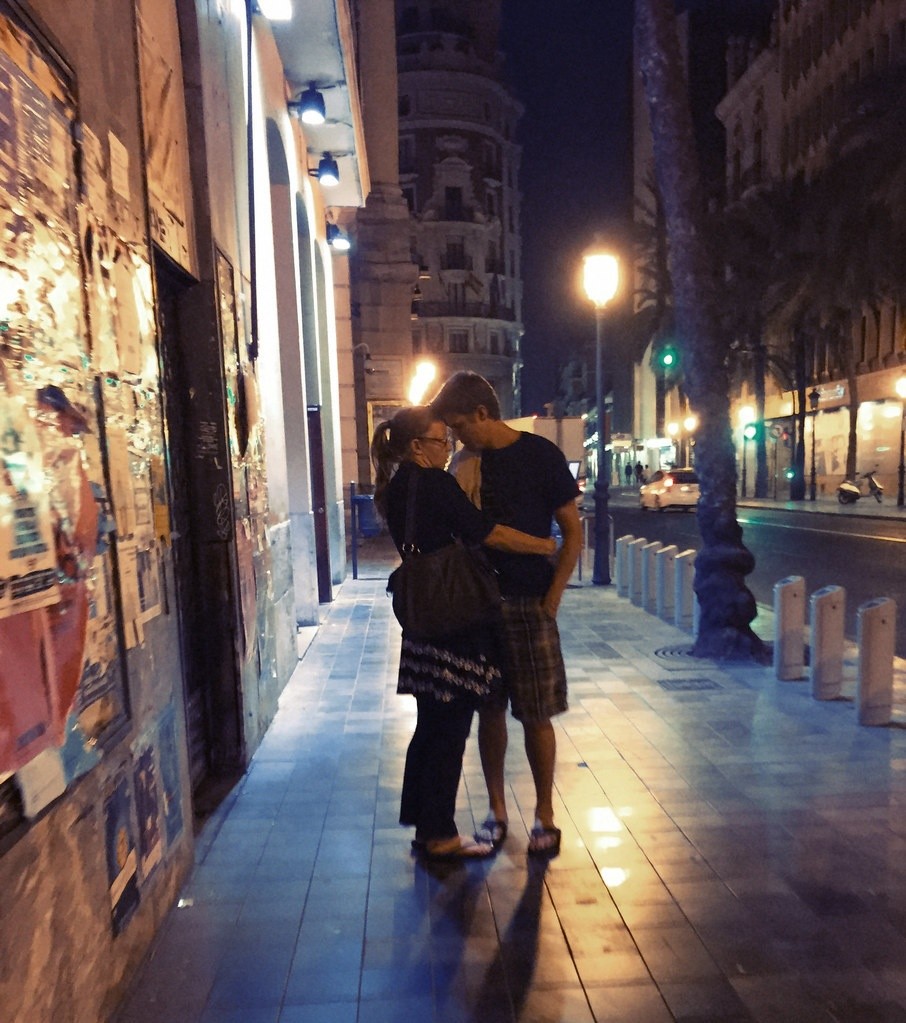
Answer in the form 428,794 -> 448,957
528,828 -> 561,857
411,831 -> 497,861
473,816 -> 508,846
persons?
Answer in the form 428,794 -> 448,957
635,461 -> 643,483
371,406 -> 557,862
641,465 -> 651,482
430,371 -> 583,854
625,462 -> 632,485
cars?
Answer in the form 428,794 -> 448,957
640,468 -> 699,511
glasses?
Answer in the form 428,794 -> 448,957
409,435 -> 448,447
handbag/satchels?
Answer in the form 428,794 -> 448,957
386,467 -> 511,639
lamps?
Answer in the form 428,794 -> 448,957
412,284 -> 424,301
308,150 -> 340,187
419,264 -> 431,281
326,223 -> 351,252
352,342 -> 376,376
286,80 -> 328,126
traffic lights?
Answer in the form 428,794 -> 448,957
745,426 -> 758,441
782,427 -> 788,447
661,346 -> 674,368
784,469 -> 794,479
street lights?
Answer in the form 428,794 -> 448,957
667,417 -> 697,467
582,231 -> 618,586
808,387 -> 821,501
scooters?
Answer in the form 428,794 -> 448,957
836,462 -> 884,504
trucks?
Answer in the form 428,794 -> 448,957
455,417 -> 586,507
